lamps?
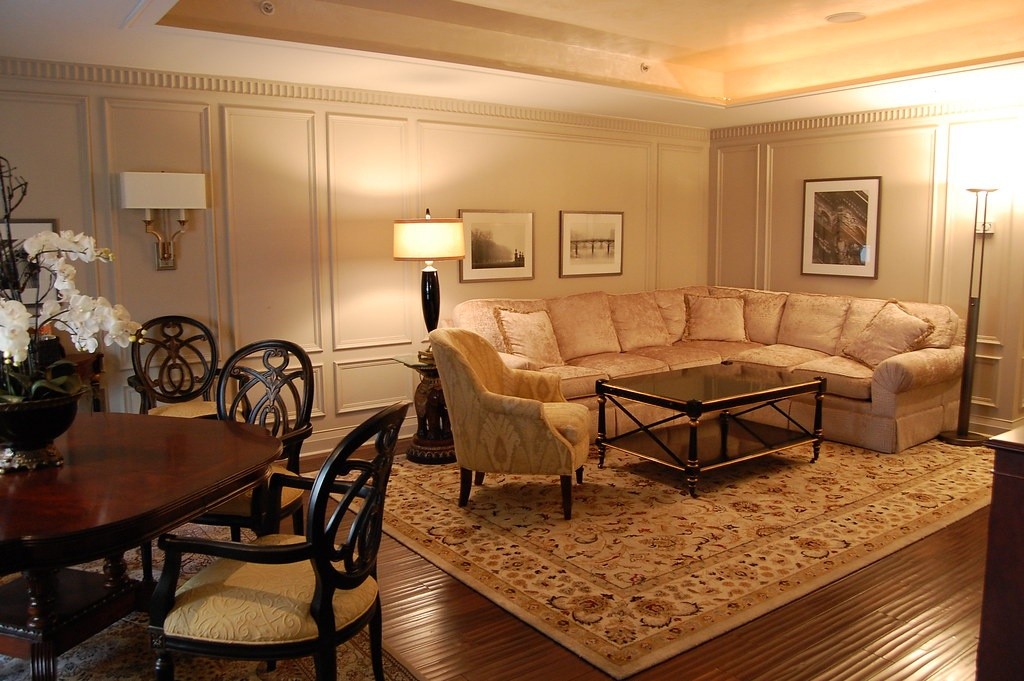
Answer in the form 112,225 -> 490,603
937,187 -> 999,446
392,208 -> 466,365
120,171 -> 207,271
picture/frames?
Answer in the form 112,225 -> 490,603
559,209 -> 624,278
801,176 -> 882,280
459,208 -> 536,284
0,217 -> 64,308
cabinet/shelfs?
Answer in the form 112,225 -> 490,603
975,423 -> 1024,681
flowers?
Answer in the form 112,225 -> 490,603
0,213 -> 146,408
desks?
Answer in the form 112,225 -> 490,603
393,353 -> 457,465
0,410 -> 284,681
64,353 -> 105,413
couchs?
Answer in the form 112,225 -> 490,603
450,284 -> 966,455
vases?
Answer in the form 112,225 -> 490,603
0,394 -> 80,475
37,334 -> 66,372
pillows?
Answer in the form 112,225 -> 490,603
490,305 -> 567,373
682,293 -> 749,344
840,296 -> 936,371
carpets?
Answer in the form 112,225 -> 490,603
304,425 -> 995,681
0,520 -> 432,681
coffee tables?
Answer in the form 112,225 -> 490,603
596,360 -> 827,497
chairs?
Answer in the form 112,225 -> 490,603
427,327 -> 590,520
152,400 -> 411,681
133,339 -> 315,596
127,315 -> 254,428
